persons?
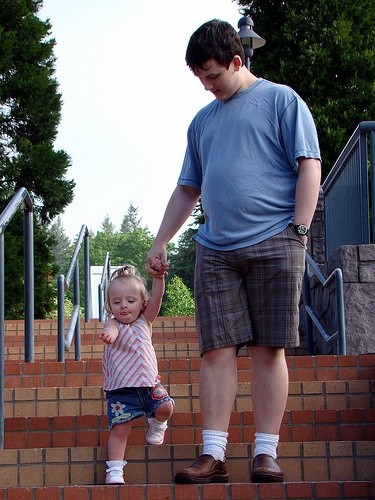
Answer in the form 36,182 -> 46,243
145,19 -> 322,484
99,258 -> 175,485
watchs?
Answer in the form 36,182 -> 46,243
294,224 -> 310,237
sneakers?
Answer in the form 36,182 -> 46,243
146,418 -> 168,444
105,466 -> 124,484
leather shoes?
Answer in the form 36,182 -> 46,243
174,454 -> 229,483
251,454 -> 284,481
